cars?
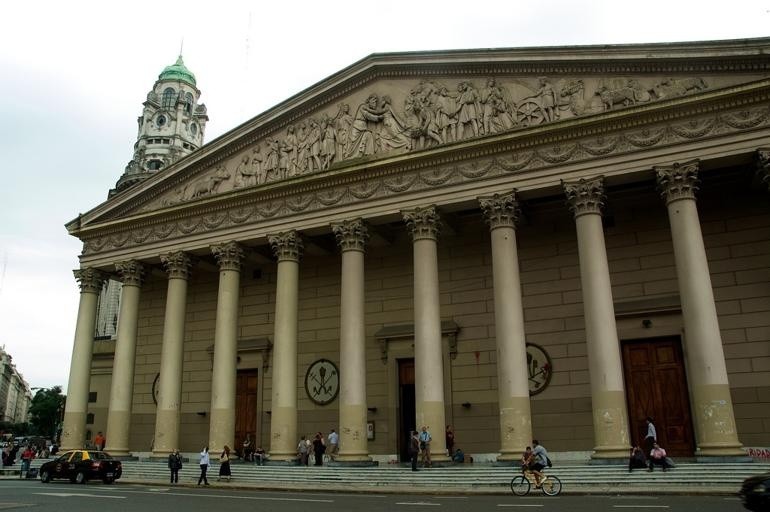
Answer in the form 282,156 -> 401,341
736,471 -> 770,511
0,431 -> 58,452
38,449 -> 122,485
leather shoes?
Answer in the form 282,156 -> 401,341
412,468 -> 420,471
648,469 -> 653,471
663,468 -> 666,472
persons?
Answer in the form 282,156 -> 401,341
297,429 -> 338,466
95,431 -> 103,451
0,435 -> 58,464
197,446 -> 211,485
522,446 -> 536,480
217,445 -> 231,483
410,431 -> 419,471
454,454 -> 473,462
629,443 -> 648,473
235,75 -> 559,188
643,417 -> 657,460
524,440 -> 549,489
419,426 -> 433,468
168,447 -> 182,483
240,434 -> 265,463
446,425 -> 454,456
648,441 -> 667,472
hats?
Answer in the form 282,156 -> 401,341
647,416 -> 653,423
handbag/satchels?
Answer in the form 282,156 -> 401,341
420,442 -> 426,450
546,457 -> 552,467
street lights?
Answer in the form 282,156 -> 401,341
31,387 -> 51,392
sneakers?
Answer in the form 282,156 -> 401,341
541,477 -> 547,483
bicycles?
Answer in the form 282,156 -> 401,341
510,461 -> 562,496
